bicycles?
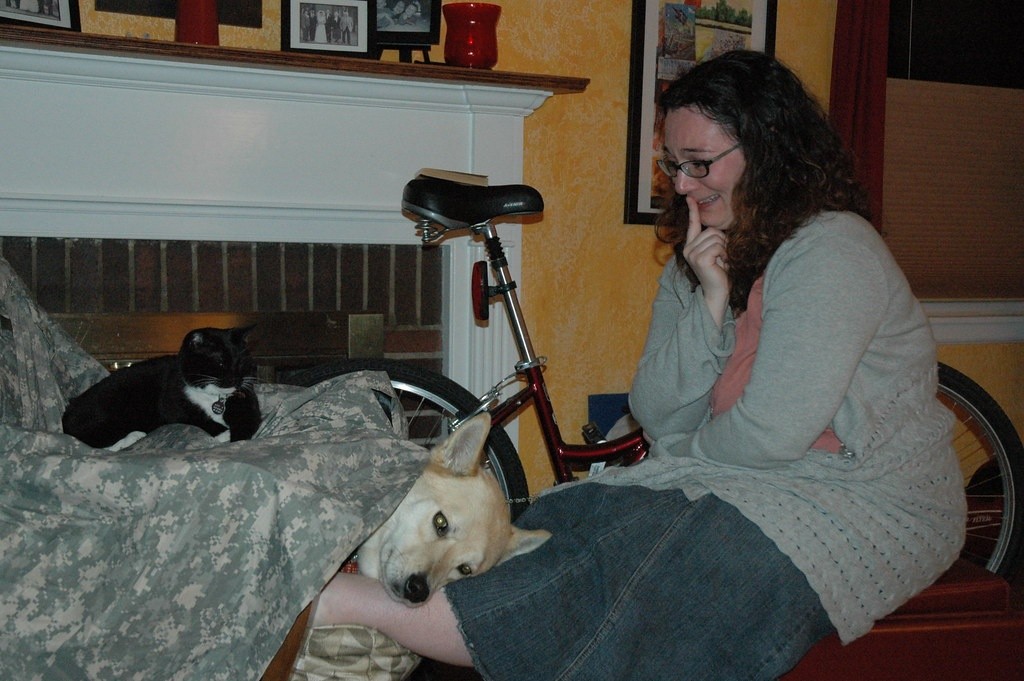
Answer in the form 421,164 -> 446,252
281,174 -> 1022,581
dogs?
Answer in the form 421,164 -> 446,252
347,412 -> 552,607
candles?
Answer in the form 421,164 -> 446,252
442,2 -> 501,69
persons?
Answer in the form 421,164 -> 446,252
311,48 -> 969,681
300,8 -> 357,46
377,0 -> 427,31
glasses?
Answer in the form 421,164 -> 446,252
655,143 -> 740,178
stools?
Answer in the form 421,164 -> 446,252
778,560 -> 1024,681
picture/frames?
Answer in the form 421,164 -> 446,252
377,0 -> 442,45
281,0 -> 378,60
0,0 -> 81,32
623,0 -> 778,228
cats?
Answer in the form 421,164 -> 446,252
61,324 -> 264,451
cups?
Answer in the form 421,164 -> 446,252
443,3 -> 502,68
